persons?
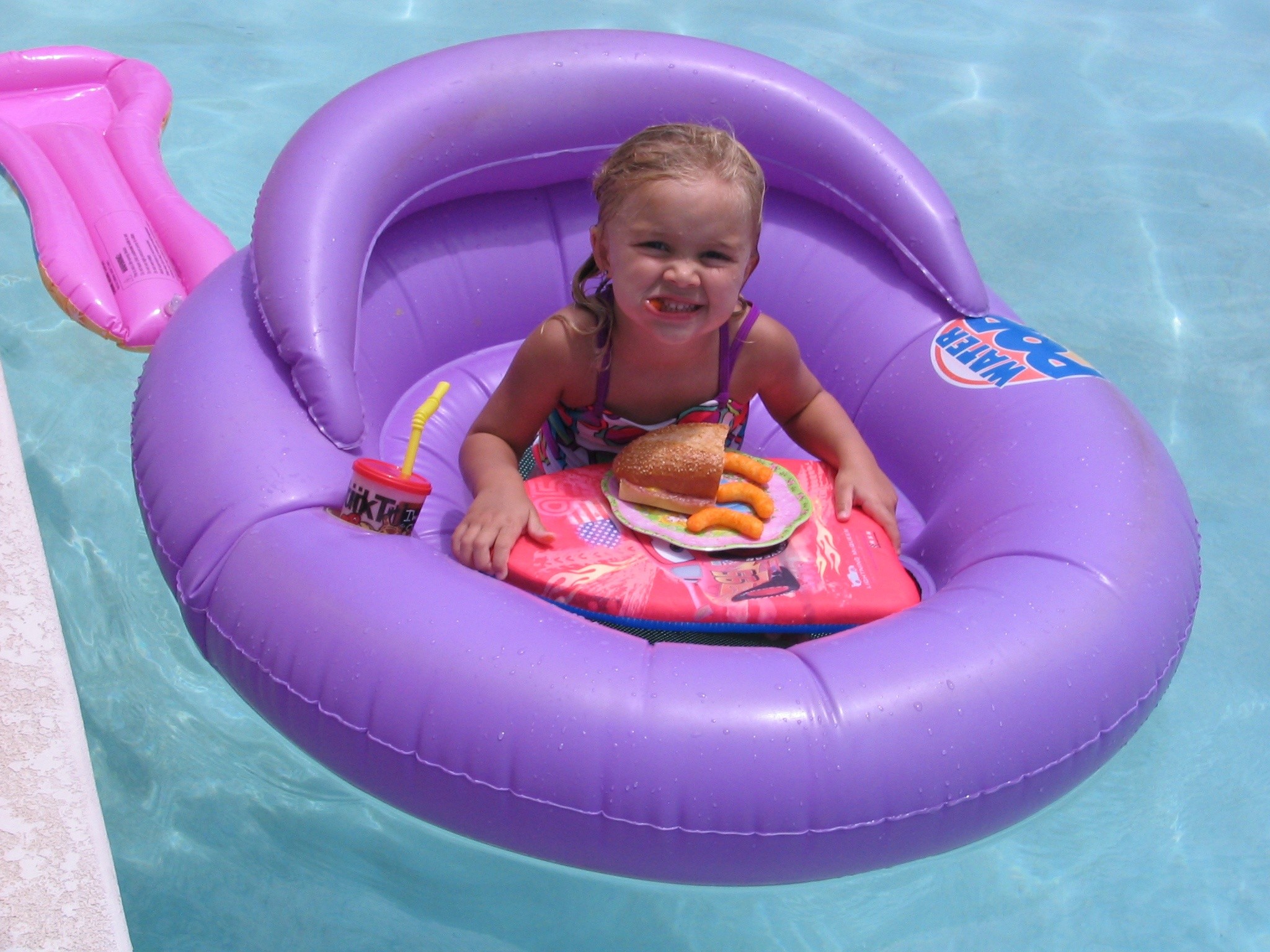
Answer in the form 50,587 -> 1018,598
449,117 -> 905,581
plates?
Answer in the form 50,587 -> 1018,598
601,449 -> 813,553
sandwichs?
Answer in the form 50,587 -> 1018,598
612,422 -> 729,515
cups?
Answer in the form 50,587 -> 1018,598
337,457 -> 433,538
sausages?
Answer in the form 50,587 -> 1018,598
724,452 -> 773,484
686,508 -> 764,539
717,482 -> 774,519
